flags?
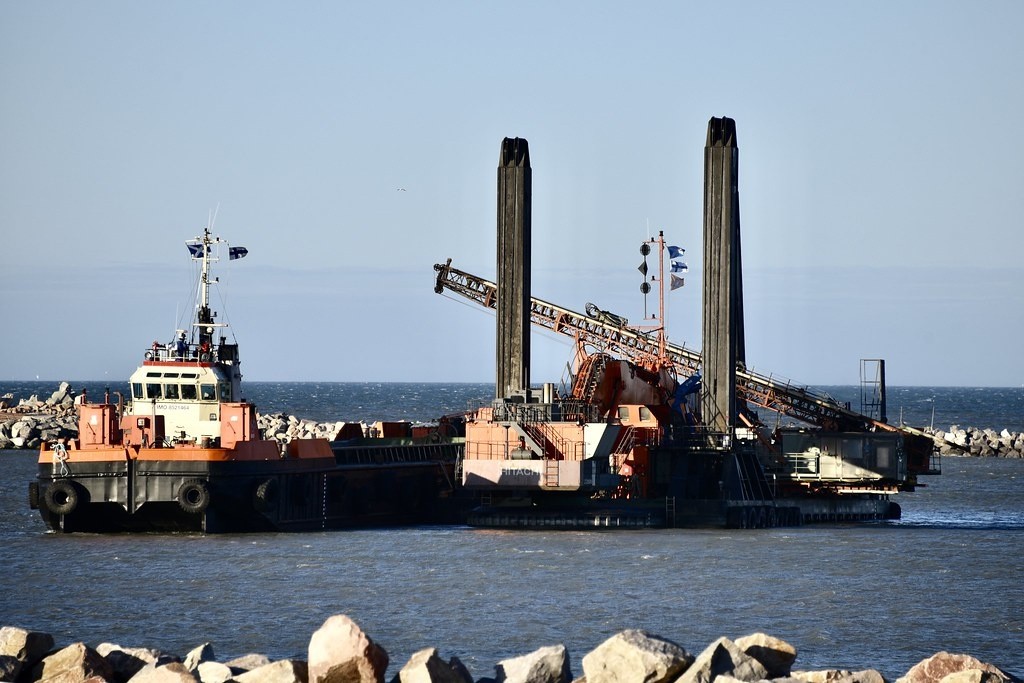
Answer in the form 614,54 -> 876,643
187,244 -> 208,258
667,246 -> 685,259
670,260 -> 689,272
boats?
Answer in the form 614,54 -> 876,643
24,115 -> 944,538
